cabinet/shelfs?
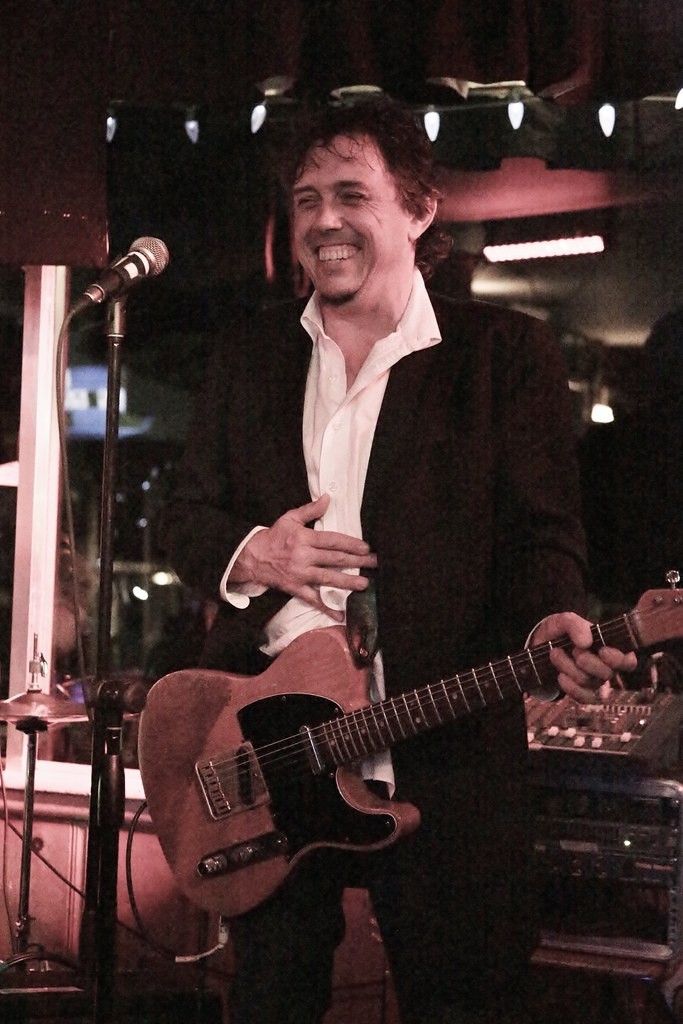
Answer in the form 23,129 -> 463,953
0,756 -> 398,1024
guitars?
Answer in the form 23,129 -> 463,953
136,569 -> 683,921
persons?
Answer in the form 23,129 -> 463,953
161,90 -> 639,1024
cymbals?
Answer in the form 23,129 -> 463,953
0,694 -> 140,727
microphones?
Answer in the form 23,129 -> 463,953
69,236 -> 169,317
